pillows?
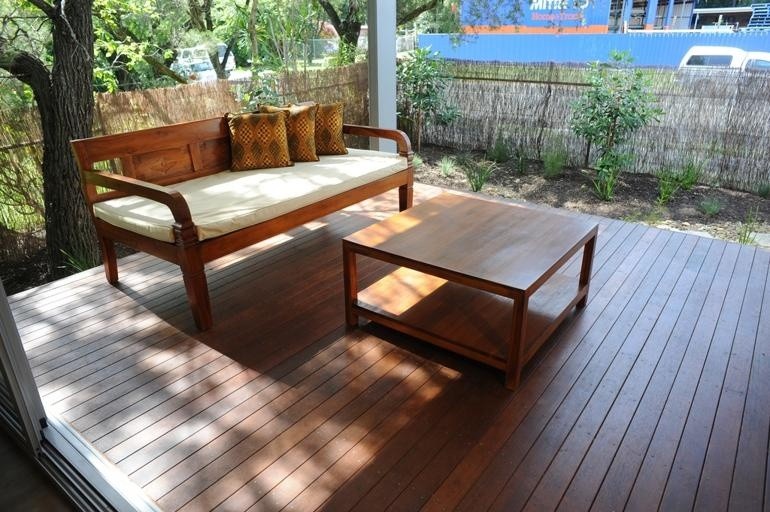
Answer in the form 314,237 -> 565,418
315,102 -> 348,155
224,110 -> 294,172
257,104 -> 320,161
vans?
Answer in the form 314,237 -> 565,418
678,46 -> 770,72
175,42 -> 236,78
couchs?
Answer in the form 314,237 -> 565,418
68,110 -> 413,332
